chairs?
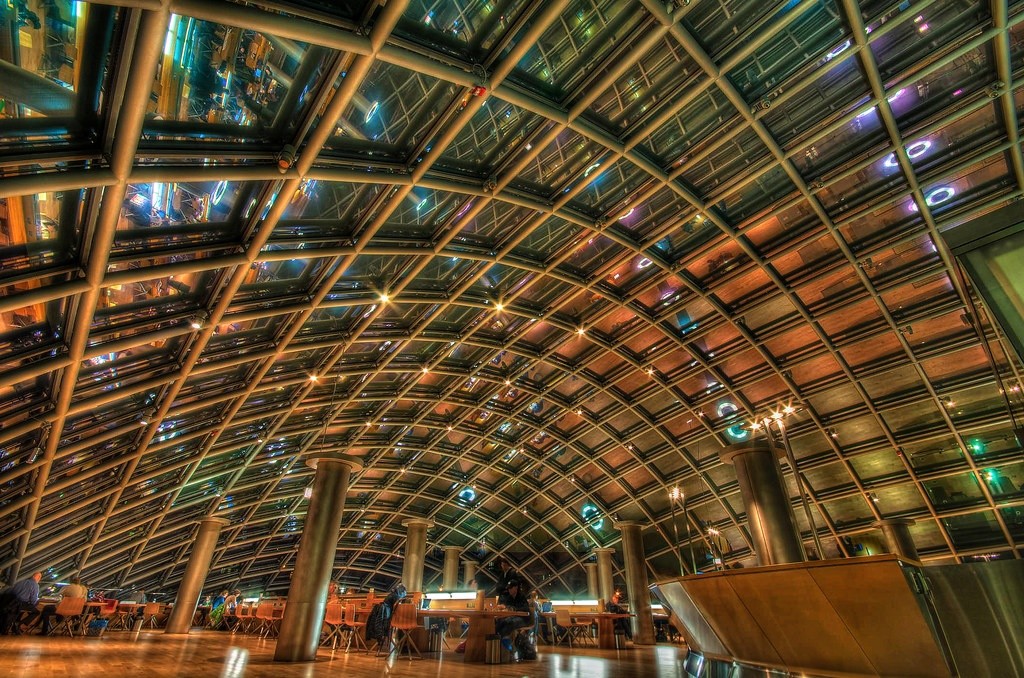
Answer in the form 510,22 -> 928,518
12,584 -> 677,659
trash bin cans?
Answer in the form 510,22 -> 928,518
130,615 -> 145,632
614,629 -> 627,650
86,619 -> 110,637
485,634 -> 502,664
430,628 -> 443,652
340,626 -> 354,649
592,623 -> 598,638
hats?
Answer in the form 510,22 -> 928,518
504,579 -> 518,589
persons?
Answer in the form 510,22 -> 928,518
493,579 -> 533,639
606,596 -> 632,639
382,585 -> 409,654
131,588 -> 149,615
500,560 -> 522,604
528,591 -> 553,645
35,574 -> 88,634
13,572 -> 44,633
208,588 -> 242,631
